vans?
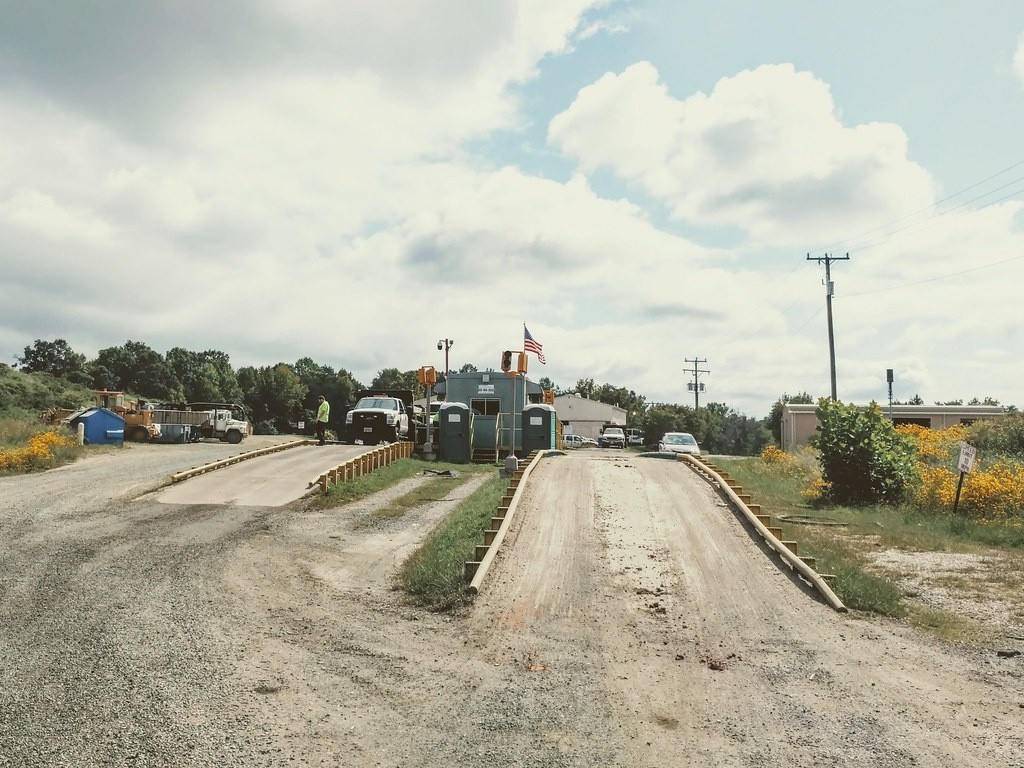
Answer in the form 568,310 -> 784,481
426,400 -> 444,443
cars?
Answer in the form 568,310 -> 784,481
561,433 -> 598,448
658,432 -> 702,458
627,429 -> 645,447
600,428 -> 626,449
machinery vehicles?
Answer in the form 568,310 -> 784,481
94,387 -> 160,445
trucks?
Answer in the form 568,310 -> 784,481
135,398 -> 254,444
344,389 -> 427,445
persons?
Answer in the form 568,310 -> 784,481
315,395 -> 330,446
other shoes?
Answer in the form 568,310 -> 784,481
316,443 -> 325,446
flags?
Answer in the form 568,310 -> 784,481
525,327 -> 545,364
